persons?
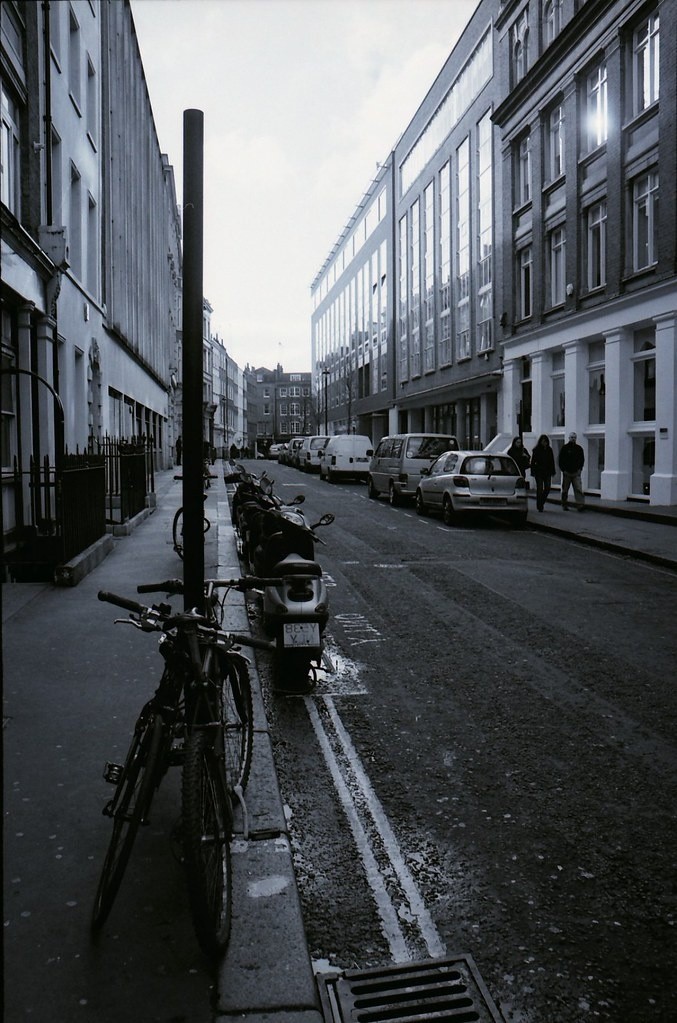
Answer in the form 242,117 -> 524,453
240,446 -> 244,459
176,436 -> 182,466
204,439 -> 217,465
229,444 -> 237,459
531,434 -> 556,512
558,432 -> 586,512
507,437 -> 531,479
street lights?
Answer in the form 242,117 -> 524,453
322,366 -> 331,436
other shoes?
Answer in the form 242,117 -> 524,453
538,505 -> 544,512
563,505 -> 569,511
578,507 -> 586,512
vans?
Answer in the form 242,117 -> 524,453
365,433 -> 460,507
318,434 -> 376,486
297,435 -> 332,473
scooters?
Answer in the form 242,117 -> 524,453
228,457 -> 305,570
241,501 -> 335,695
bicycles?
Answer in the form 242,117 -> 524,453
202,458 -> 211,489
173,475 -> 218,558
86,573 -> 285,963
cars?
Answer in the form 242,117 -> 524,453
269,444 -> 283,459
257,452 -> 264,460
278,442 -> 290,465
414,451 -> 529,529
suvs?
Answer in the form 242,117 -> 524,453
286,437 -> 304,467
